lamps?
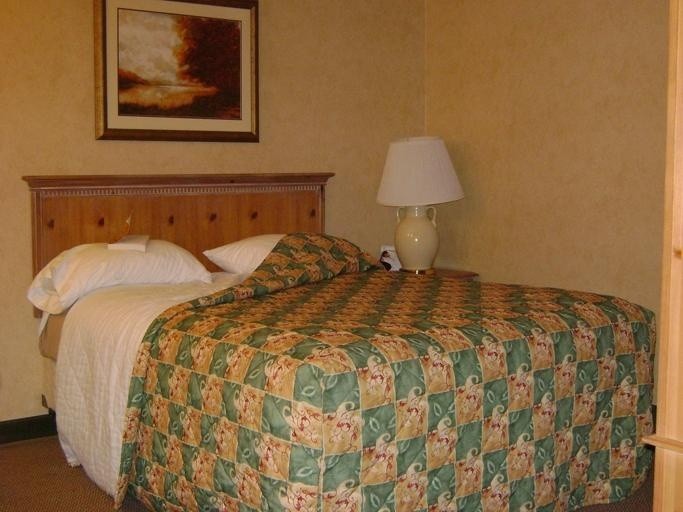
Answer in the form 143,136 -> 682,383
375,135 -> 464,269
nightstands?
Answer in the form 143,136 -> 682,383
406,268 -> 479,282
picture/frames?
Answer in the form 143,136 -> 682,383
91,1 -> 259,144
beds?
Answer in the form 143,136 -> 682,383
17,171 -> 657,511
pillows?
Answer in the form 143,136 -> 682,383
26,237 -> 211,316
201,233 -> 363,275
